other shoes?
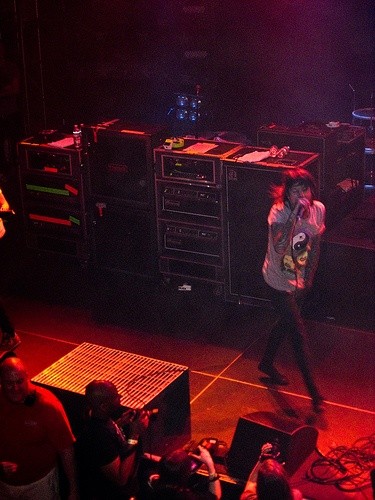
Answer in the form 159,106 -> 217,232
0,332 -> 22,360
310,392 -> 325,415
258,364 -> 289,385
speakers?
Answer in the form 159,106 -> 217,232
227,410 -> 318,481
88,199 -> 160,285
222,146 -> 321,304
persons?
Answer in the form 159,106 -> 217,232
0,189 -> 21,352
240,443 -> 303,500
257,167 -> 327,406
0,357 -> 76,500
70,379 -> 149,500
148,445 -> 222,500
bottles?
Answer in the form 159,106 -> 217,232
72,125 -> 81,148
278,146 -> 291,158
79,123 -> 85,145
270,145 -> 278,157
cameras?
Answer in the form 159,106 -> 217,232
261,440 -> 280,462
132,409 -> 160,422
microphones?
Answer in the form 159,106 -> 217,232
296,206 -> 306,220
